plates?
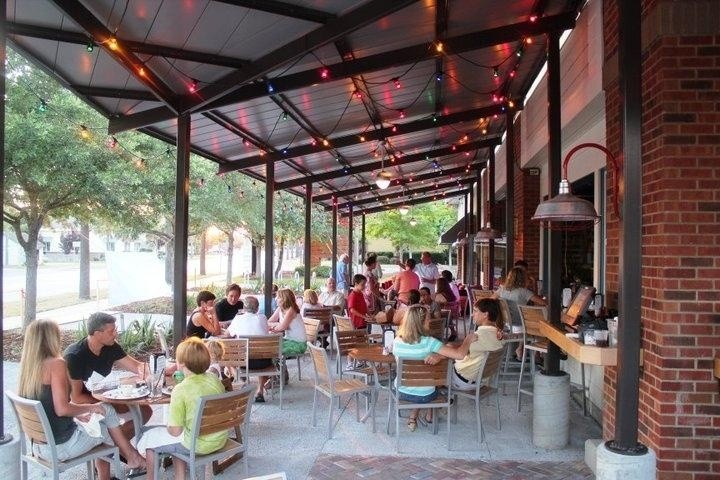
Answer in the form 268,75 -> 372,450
91,370 -> 185,402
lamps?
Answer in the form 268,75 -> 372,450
399,185 -> 409,216
376,140 -> 391,191
409,204 -> 417,226
451,227 -> 518,249
526,142 -> 624,232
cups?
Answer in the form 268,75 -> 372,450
582,328 -> 598,345
594,329 -> 610,348
384,331 -> 395,354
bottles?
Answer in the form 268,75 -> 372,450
562,283 -> 572,307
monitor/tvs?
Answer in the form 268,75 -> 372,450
562,285 -> 596,325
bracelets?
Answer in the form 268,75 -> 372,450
273,325 -> 276,330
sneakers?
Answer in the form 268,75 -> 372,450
345,363 -> 364,371
126,460 -> 147,478
254,393 -> 266,403
407,419 -> 418,432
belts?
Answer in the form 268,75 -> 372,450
453,369 -> 484,386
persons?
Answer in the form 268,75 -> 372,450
216,282 -> 242,322
185,291 -> 220,339
18,317 -> 147,480
300,289 -> 321,318
268,289 -> 308,385
66,313 -> 152,463
259,284 -> 277,316
137,337 -> 227,479
207,341 -> 225,380
224,296 -> 271,402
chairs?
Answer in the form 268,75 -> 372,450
91,338 -> 258,480
245,471 -> 286,479
5,390 -> 121,479
303,282 -> 552,453
204,317 -> 320,410
